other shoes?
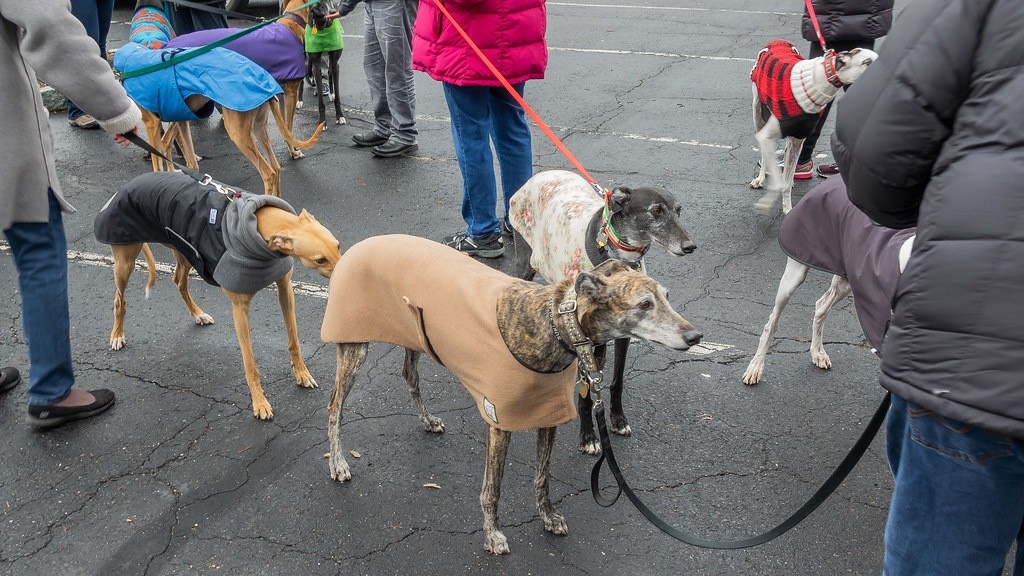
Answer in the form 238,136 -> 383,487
68,115 -> 99,128
314,83 -> 329,96
28,389 -> 115,425
0,367 -> 21,393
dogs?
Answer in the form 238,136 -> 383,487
95,171 -> 343,421
326,232 -> 705,555
752,40 -> 879,216
510,168 -> 698,456
108,0 -> 327,197
741,173 -> 913,385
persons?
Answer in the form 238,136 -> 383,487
411,0 -> 548,257
325,0 -> 419,157
68,0 -> 114,128
0,0 -> 142,427
829,0 -> 1024,576
757,0 -> 894,179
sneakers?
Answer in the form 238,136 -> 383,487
498,216 -> 514,236
758,157 -> 814,178
816,162 -> 840,178
443,232 -> 505,257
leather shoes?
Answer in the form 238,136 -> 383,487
351,129 -> 388,145
372,140 -> 418,157
